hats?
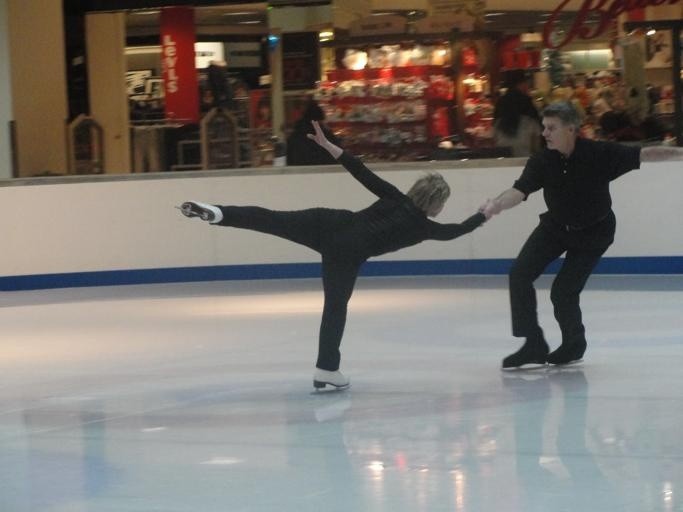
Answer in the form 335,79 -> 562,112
499,69 -> 532,88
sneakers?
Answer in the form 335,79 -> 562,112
181,201 -> 224,224
313,369 -> 349,388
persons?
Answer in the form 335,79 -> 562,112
477,100 -> 683,368
181,119 -> 503,390
492,69 -> 544,158
286,105 -> 345,165
503,372 -> 617,500
568,82 -> 659,132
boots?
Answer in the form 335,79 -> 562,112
502,328 -> 548,368
547,326 -> 587,364
